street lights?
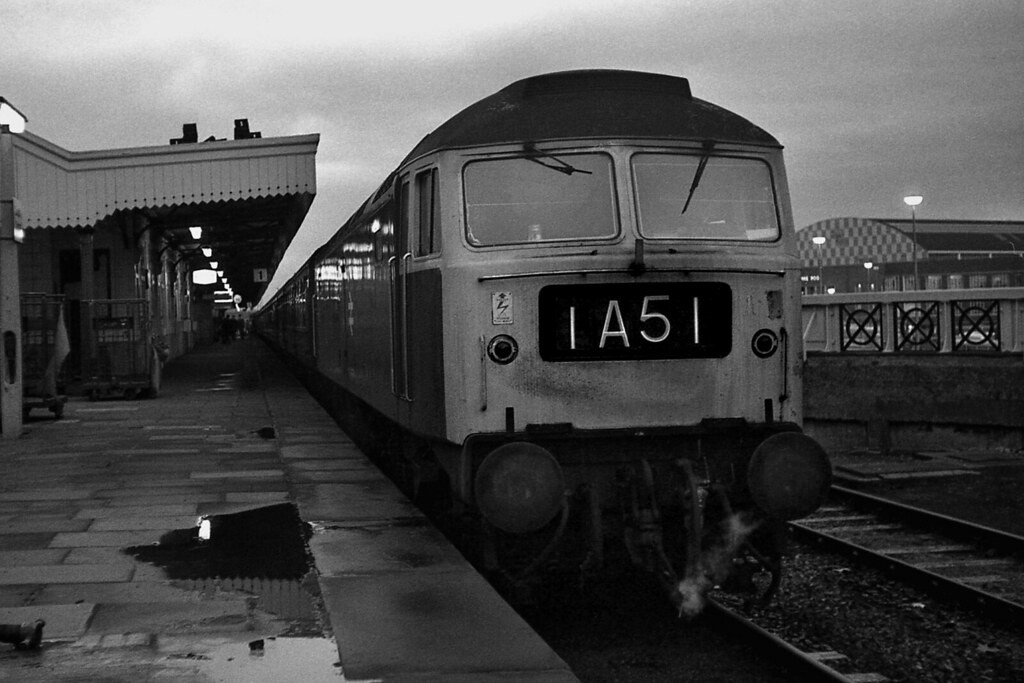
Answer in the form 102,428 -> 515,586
903,195 -> 923,351
864,261 -> 874,326
812,237 -> 827,294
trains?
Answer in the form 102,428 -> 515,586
248,69 -> 832,624
